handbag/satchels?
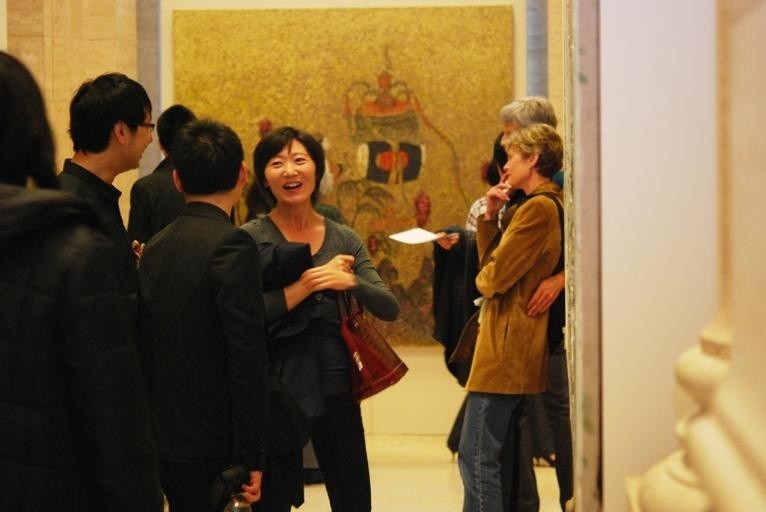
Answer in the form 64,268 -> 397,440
342,312 -> 408,402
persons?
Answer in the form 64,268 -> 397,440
435,97 -> 573,512
246,176 -> 341,225
128,105 -> 234,243
0,52 -> 171,512
237,129 -> 400,512
138,119 -> 311,512
57,73 -> 154,385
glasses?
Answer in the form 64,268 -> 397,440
137,122 -> 155,131
